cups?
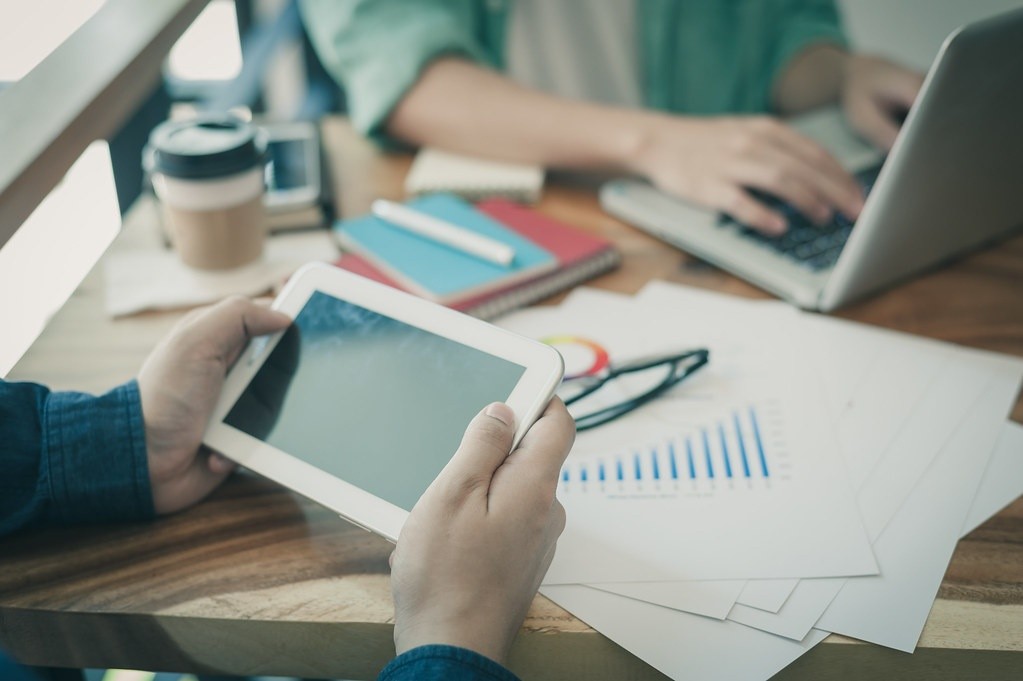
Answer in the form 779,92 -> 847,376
141,112 -> 266,271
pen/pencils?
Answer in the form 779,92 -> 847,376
369,200 -> 512,269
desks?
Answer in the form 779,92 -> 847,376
0,116 -> 1023,681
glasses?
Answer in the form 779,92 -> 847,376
564,348 -> 709,432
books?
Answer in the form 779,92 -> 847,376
404,145 -> 549,205
332,191 -> 622,321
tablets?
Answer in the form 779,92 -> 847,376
201,260 -> 565,544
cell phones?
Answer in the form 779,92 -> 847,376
263,126 -> 322,217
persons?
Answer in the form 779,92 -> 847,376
297,2 -> 929,238
0,296 -> 575,681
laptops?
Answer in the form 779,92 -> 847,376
600,4 -> 1023,315
